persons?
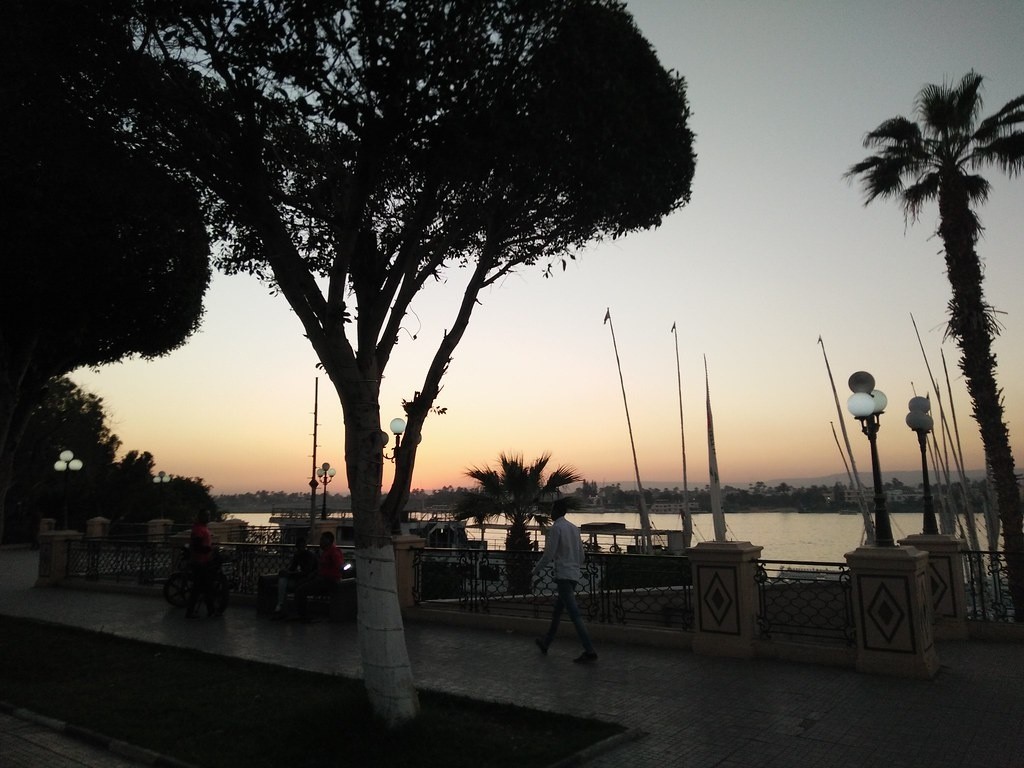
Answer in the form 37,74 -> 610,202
529,503 -> 598,663
184,509 -> 225,622
291,531 -> 344,623
265,537 -> 314,621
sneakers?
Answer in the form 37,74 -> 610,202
535,639 -> 547,653
573,650 -> 597,664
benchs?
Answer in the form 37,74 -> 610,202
257,573 -> 357,622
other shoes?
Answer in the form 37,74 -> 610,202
184,612 -> 200,620
